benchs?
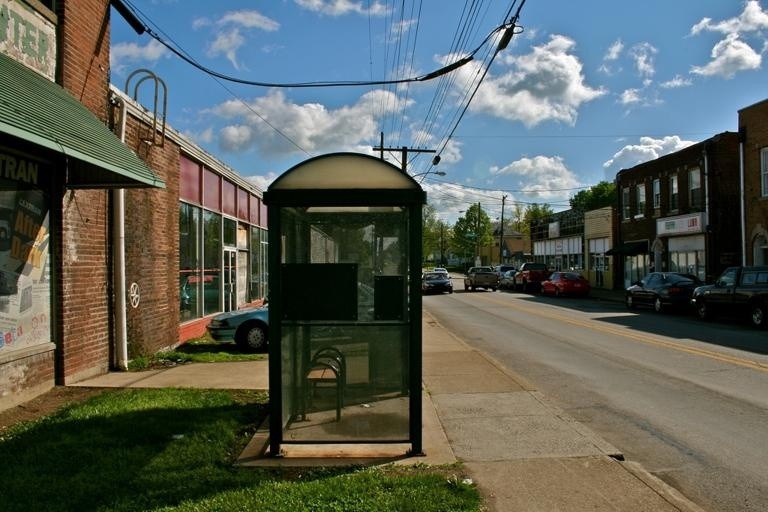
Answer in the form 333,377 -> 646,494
302,348 -> 346,422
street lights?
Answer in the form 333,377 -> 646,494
411,171 -> 446,179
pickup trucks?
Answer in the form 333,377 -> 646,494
464,266 -> 498,292
513,262 -> 552,292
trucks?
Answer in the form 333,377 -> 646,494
693,265 -> 768,327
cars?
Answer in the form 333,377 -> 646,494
206,280 -> 374,354
181,269 -> 234,312
540,271 -> 592,298
625,272 -> 704,313
493,264 -> 519,290
422,268 -> 453,294
